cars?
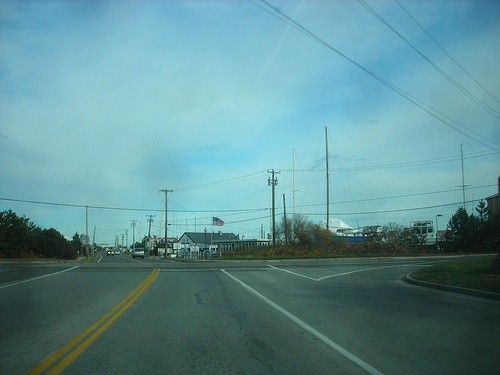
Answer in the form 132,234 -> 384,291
115,250 -> 120,255
107,250 -> 114,256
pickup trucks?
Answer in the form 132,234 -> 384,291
132,248 -> 145,259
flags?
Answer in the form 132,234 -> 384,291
212,216 -> 225,227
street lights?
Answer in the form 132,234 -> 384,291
203,227 -> 208,259
436,214 -> 443,251
